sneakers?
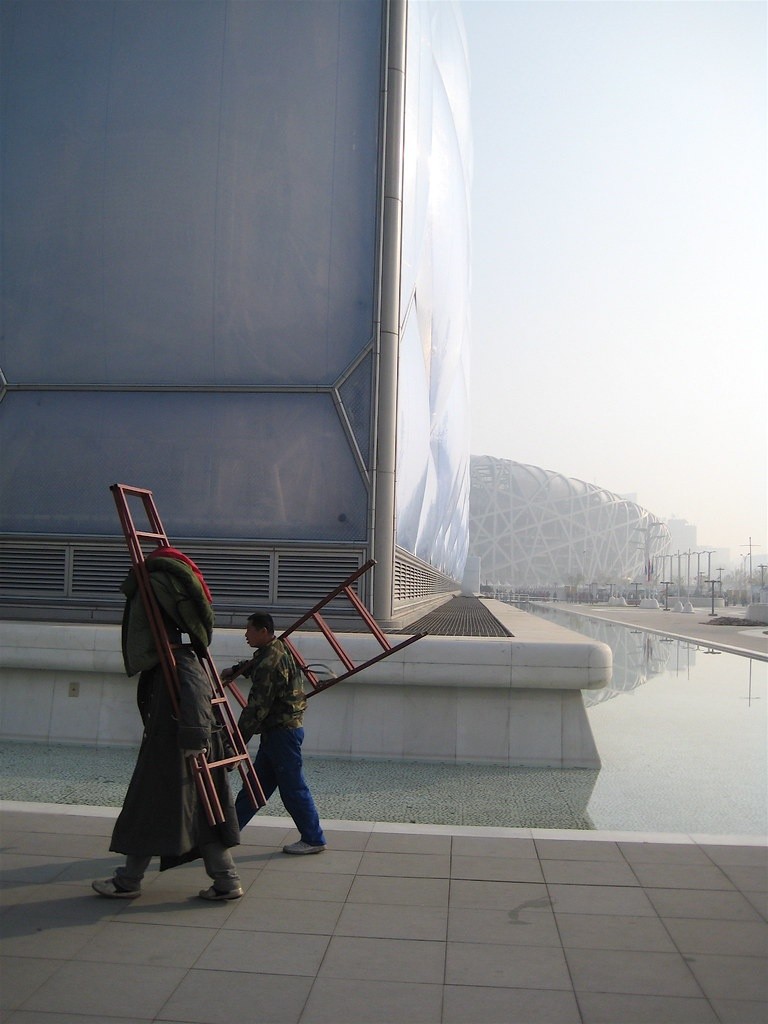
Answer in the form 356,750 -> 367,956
283,840 -> 328,854
199,886 -> 244,900
92,878 -> 143,898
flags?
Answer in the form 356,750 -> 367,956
645,557 -> 654,582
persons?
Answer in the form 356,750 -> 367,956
495,589 -> 760,607
91,629 -> 244,901
219,614 -> 326,854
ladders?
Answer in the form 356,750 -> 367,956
215,555 -> 431,735
109,482 -> 269,827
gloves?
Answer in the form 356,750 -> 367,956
184,747 -> 208,758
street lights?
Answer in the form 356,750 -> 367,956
703,551 -> 716,595
630,582 -> 643,607
655,552 -> 686,598
740,553 -> 750,606
683,547 -> 694,597
758,564 -> 767,589
716,568 -> 724,597
692,551 -> 703,595
704,580 -> 720,616
606,583 -> 616,599
660,582 -> 675,611
